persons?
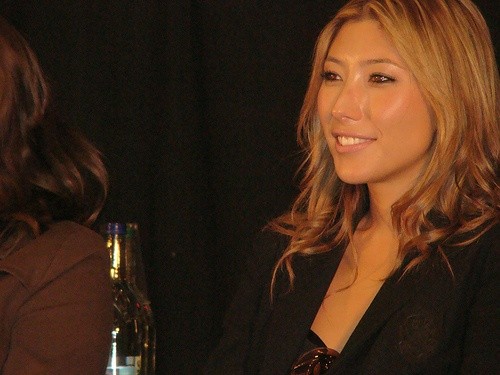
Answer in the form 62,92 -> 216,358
211,1 -> 499,375
0,16 -> 114,375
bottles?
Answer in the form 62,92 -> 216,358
96,221 -> 150,374
122,223 -> 158,375
